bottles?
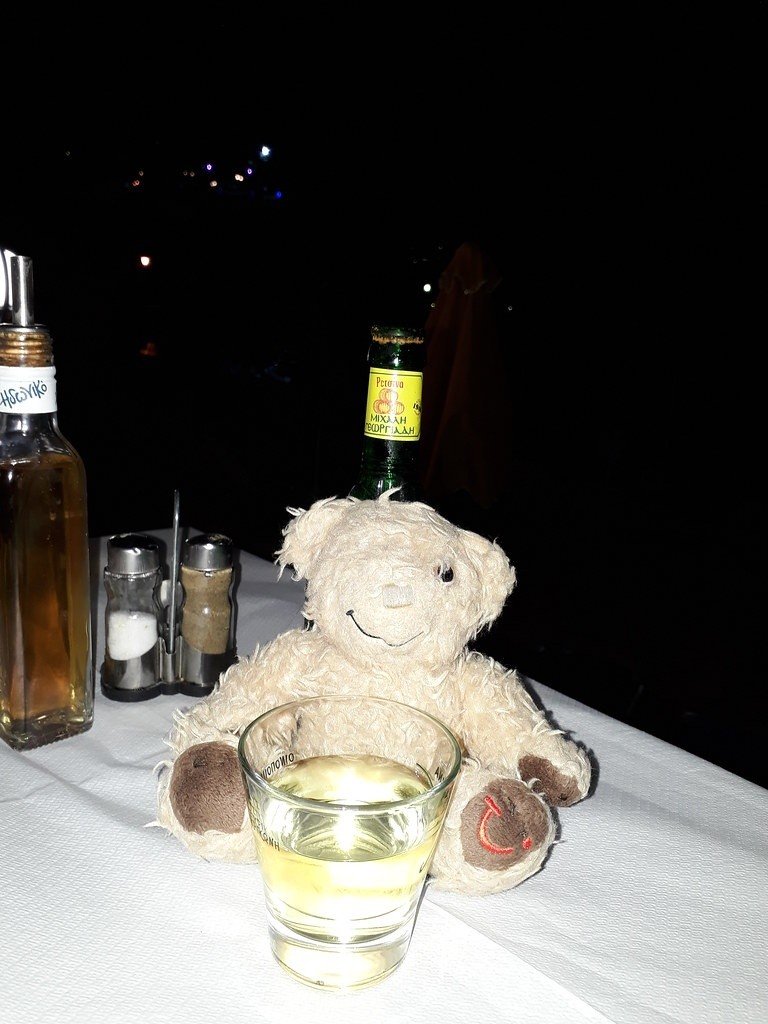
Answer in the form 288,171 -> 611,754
0,324 -> 94,753
342,324 -> 429,502
100,533 -> 163,688
179,533 -> 241,654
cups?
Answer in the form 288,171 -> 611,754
236,692 -> 460,994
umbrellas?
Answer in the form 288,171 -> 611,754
416,237 -> 515,510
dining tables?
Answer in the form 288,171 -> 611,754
0,526 -> 766,1021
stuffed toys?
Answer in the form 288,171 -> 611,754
157,495 -> 591,895
